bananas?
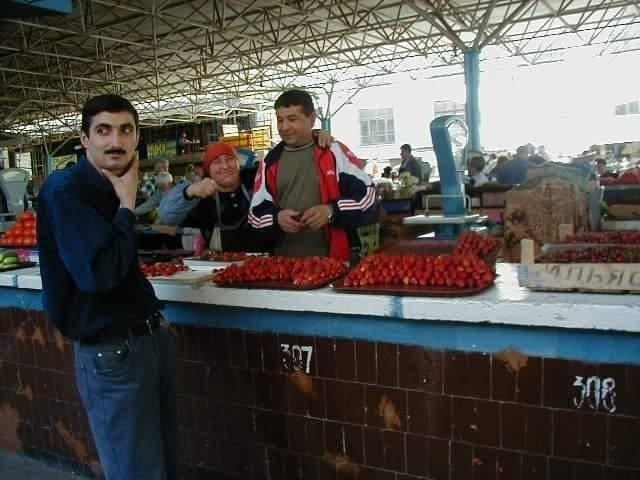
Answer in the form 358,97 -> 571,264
0,251 -> 20,269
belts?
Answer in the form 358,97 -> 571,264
80,311 -> 160,345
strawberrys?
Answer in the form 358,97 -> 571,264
212,231 -> 497,289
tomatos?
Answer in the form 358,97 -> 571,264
0,212 -> 39,247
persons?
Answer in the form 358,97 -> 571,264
159,126 -> 335,254
244,86 -> 377,263
30,92 -> 183,478
456,140 -> 620,181
398,142 -> 423,183
129,154 -> 206,227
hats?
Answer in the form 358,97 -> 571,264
204,142 -> 235,175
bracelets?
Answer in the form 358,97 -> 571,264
328,204 -> 335,224
